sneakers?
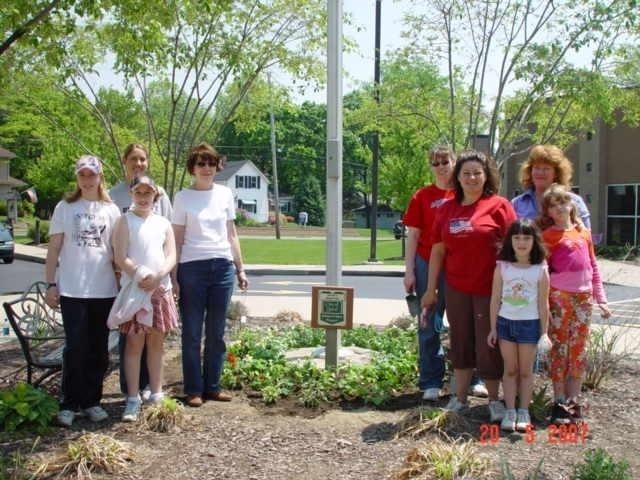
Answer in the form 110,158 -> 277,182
421,382 -> 583,432
53,387 -> 233,427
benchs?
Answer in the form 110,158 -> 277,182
4,282 -> 120,396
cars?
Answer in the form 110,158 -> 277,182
393,219 -> 409,240
0,213 -> 15,264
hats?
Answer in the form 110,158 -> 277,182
130,175 -> 157,193
75,156 -> 101,175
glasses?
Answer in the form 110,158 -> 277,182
431,159 -> 449,168
196,161 -> 217,167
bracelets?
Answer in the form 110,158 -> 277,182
48,282 -> 57,287
237,269 -> 245,273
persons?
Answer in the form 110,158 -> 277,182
403,148 -> 488,400
111,176 -> 177,420
172,142 -> 248,407
487,219 -> 550,431
46,154 -> 121,427
420,151 -> 517,412
508,146 -> 591,226
110,144 -> 174,405
535,184 -> 611,425
